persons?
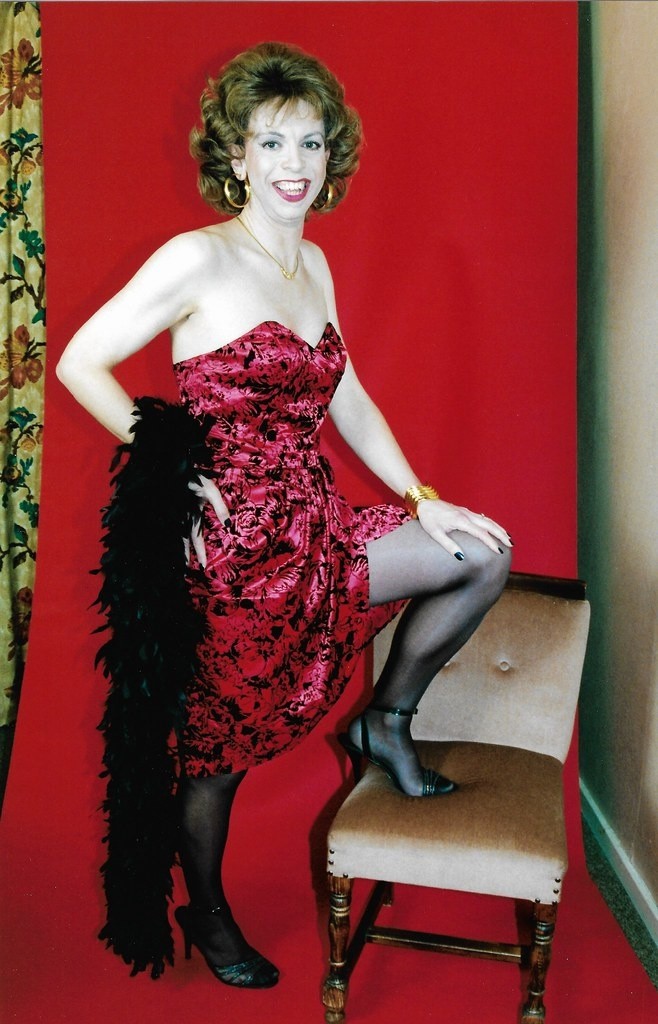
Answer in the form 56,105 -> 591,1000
52,40 -> 513,990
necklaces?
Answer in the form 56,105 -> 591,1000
237,212 -> 299,279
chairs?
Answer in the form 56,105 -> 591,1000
322,571 -> 592,1024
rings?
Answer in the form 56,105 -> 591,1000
481,513 -> 484,519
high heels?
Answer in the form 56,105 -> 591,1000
337,698 -> 456,797
174,899 -> 279,989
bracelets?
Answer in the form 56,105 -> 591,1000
404,483 -> 440,520
